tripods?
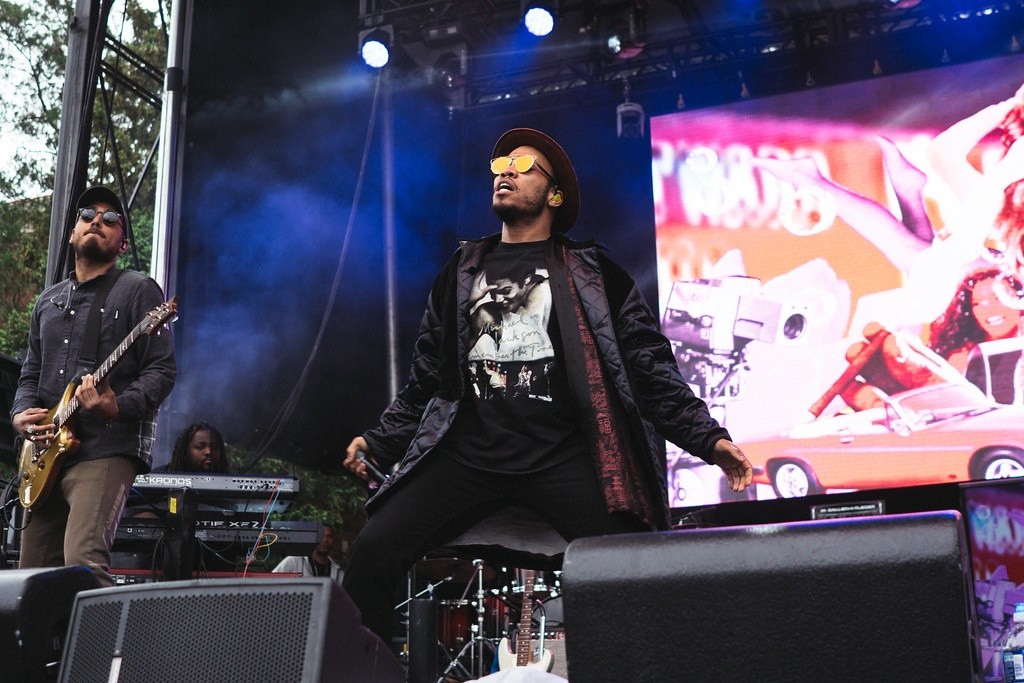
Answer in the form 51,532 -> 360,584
435,560 -> 504,683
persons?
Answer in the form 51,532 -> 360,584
152,421 -> 229,476
271,523 -> 344,586
840,322 -> 995,413
328,124 -> 754,631
11,186 -> 179,589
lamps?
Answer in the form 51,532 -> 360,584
426,41 -> 473,91
520,0 -> 561,37
617,77 -> 645,138
599,5 -> 646,61
359,13 -> 396,68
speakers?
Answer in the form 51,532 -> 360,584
562,511 -> 985,683
0,566 -> 406,683
507,630 -> 569,680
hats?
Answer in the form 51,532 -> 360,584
490,128 -> 580,232
76,186 -> 128,240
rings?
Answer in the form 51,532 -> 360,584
30,435 -> 36,441
28,426 -> 33,434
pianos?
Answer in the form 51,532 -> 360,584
108,472 -> 322,585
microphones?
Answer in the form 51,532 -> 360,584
355,449 -> 388,486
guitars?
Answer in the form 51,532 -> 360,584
497,569 -> 554,672
17,293 -> 182,512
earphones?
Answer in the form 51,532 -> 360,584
552,194 -> 561,202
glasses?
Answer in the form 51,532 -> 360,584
76,207 -> 125,237
490,155 -> 557,195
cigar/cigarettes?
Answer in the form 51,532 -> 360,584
809,328 -> 890,418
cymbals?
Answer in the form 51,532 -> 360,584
416,555 -> 496,586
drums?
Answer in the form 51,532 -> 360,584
437,597 -> 483,659
528,591 -> 564,629
510,566 -> 562,599
472,587 -> 517,644
